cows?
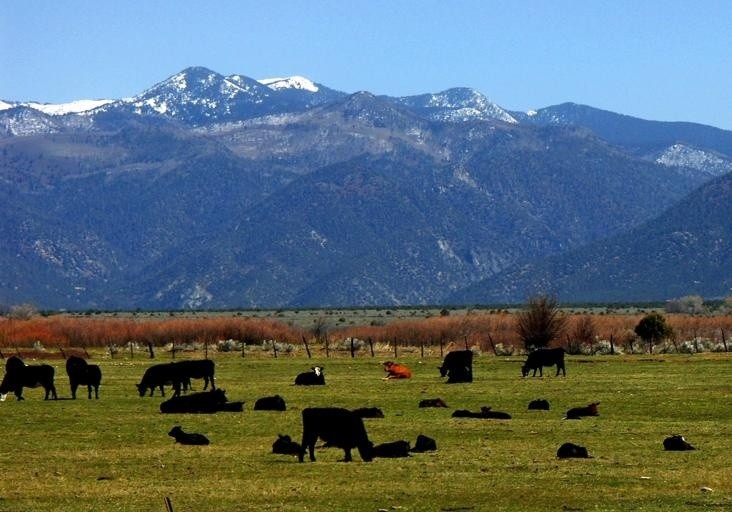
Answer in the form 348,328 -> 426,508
521,347 -> 565,377
418,398 -> 449,408
168,426 -> 210,445
557,442 -> 594,458
451,406 -> 512,419
289,365 -> 326,386
663,435 -> 696,451
135,359 -> 246,413
0,356 -> 102,401
437,350 -> 473,384
567,402 -> 600,419
528,399 -> 549,410
272,407 -> 437,463
379,361 -> 412,381
254,395 -> 286,411
